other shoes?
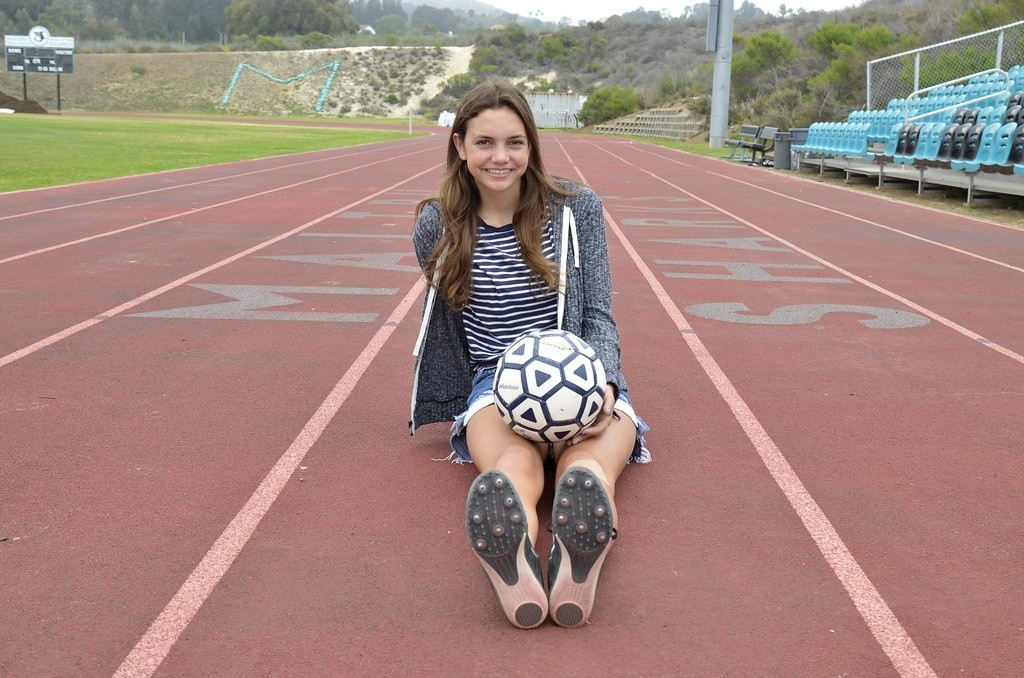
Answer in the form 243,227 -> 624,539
465,470 -> 547,630
546,461 -> 618,628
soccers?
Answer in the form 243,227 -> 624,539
492,326 -> 609,441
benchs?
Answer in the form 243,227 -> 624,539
724,125 -> 762,163
742,126 -> 781,165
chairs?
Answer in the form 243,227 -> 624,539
790,61 -> 1024,178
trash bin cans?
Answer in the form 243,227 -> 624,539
774,132 -> 791,170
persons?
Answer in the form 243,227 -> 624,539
408,81 -> 648,629
